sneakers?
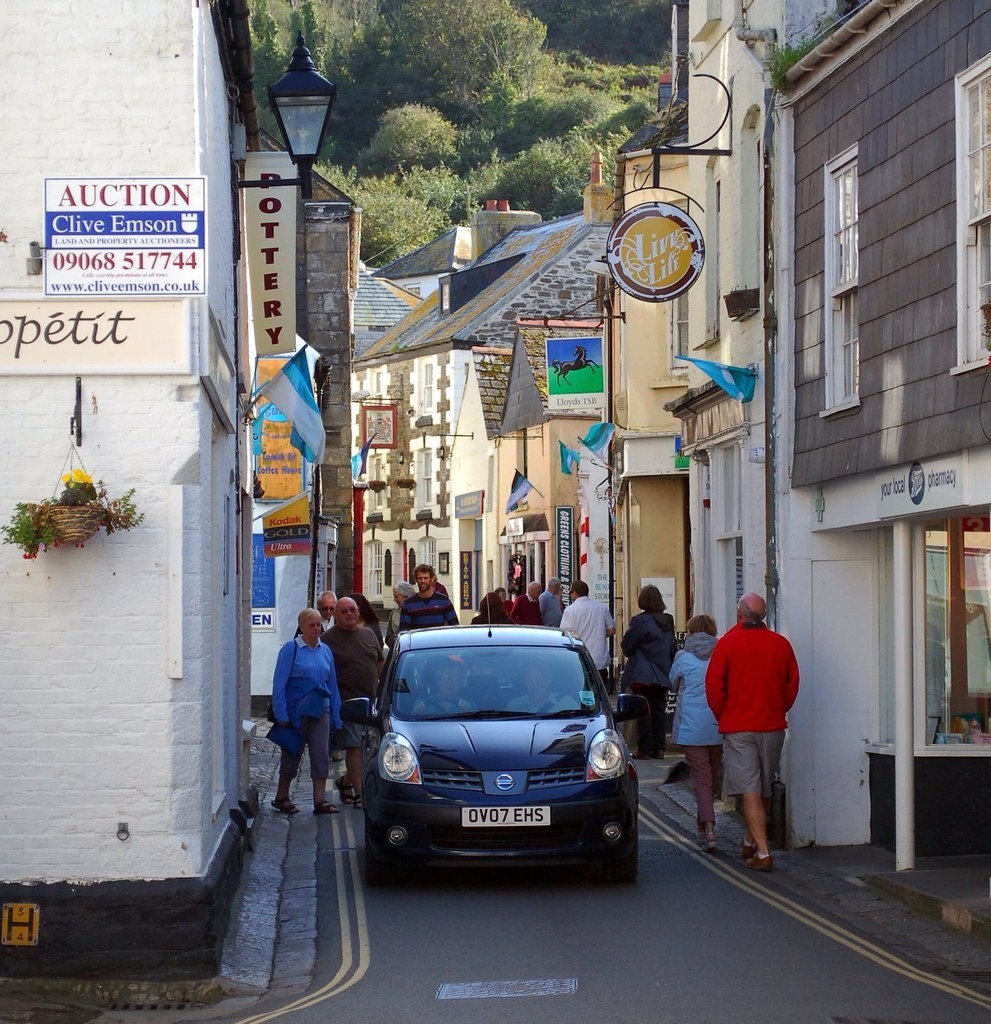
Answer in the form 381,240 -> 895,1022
631,751 -> 650,759
651,748 -> 665,759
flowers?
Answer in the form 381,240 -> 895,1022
0,469 -> 148,561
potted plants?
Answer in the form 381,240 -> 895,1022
397,479 -> 414,488
370,480 -> 385,492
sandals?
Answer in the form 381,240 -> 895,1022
352,793 -> 362,808
313,800 -> 340,814
334,776 -> 354,804
271,795 -> 299,814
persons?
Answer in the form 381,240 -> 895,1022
414,663 -> 475,715
470,578 -> 565,629
385,564 -> 459,650
264,591 -> 383,814
668,614 -> 722,849
620,584 -> 677,759
560,581 -> 616,687
705,590 -> 799,872
505,665 -> 581,712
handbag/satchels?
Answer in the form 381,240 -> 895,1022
266,724 -> 305,759
266,701 -> 277,723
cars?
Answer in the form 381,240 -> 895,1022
338,621 -> 652,887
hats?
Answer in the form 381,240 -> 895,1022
393,583 -> 415,598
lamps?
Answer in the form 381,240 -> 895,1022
236,30 -> 339,200
399,452 -> 404,464
585,262 -> 613,279
440,446 -> 445,460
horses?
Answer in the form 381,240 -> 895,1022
552,346 -> 599,386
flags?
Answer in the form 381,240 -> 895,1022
678,356 -> 755,403
558,422 -> 616,475
262,497 -> 311,556
505,471 -> 536,513
351,435 -> 377,479
265,348 -> 323,463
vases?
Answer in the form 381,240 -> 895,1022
725,288 -> 759,317
48,506 -> 98,543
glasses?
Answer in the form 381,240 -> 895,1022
318,603 -> 335,612
335,607 -> 360,615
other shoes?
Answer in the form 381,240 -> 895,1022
695,833 -> 707,844
743,854 -> 773,871
701,832 -> 717,851
331,750 -> 342,762
741,834 -> 757,857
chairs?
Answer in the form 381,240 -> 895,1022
423,657 -> 451,686
518,664 -> 576,699
466,676 -> 498,688
